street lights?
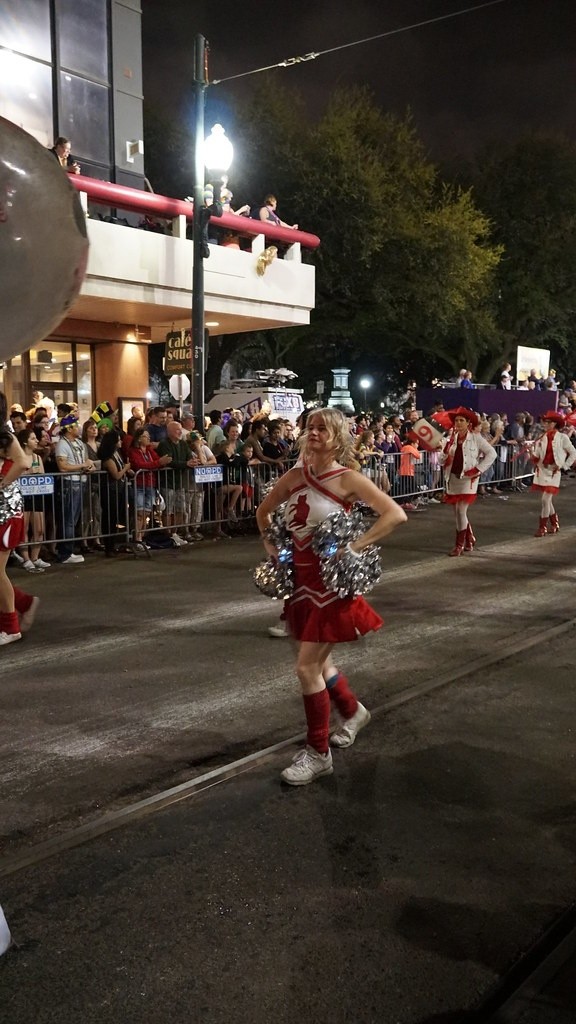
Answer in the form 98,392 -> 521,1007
360,379 -> 369,410
188,30 -> 235,432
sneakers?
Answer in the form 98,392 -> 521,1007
21,597 -> 39,632
268,618 -> 289,636
330,701 -> 371,748
281,744 -> 334,784
0,631 -> 22,645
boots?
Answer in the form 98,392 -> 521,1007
464,523 -> 476,550
450,529 -> 467,556
535,515 -> 549,537
548,513 -> 560,533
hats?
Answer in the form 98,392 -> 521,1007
58,410 -> 78,428
181,412 -> 195,420
543,410 -> 565,429
448,406 -> 478,430
97,418 -> 113,429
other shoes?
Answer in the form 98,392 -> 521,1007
62,507 -> 254,563
398,480 -> 529,510
23,558 -> 51,572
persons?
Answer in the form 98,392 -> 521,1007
49,136 -> 82,174
254,406 -> 408,785
346,400 -> 576,516
204,173 -> 250,250
0,391 -> 298,571
558,378 -> 576,414
496,362 -> 513,390
521,368 -> 560,391
259,192 -> 298,258
438,408 -> 497,555
0,391 -> 38,644
456,369 -> 473,388
532,411 -> 576,537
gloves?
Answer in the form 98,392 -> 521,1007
465,467 -> 479,476
